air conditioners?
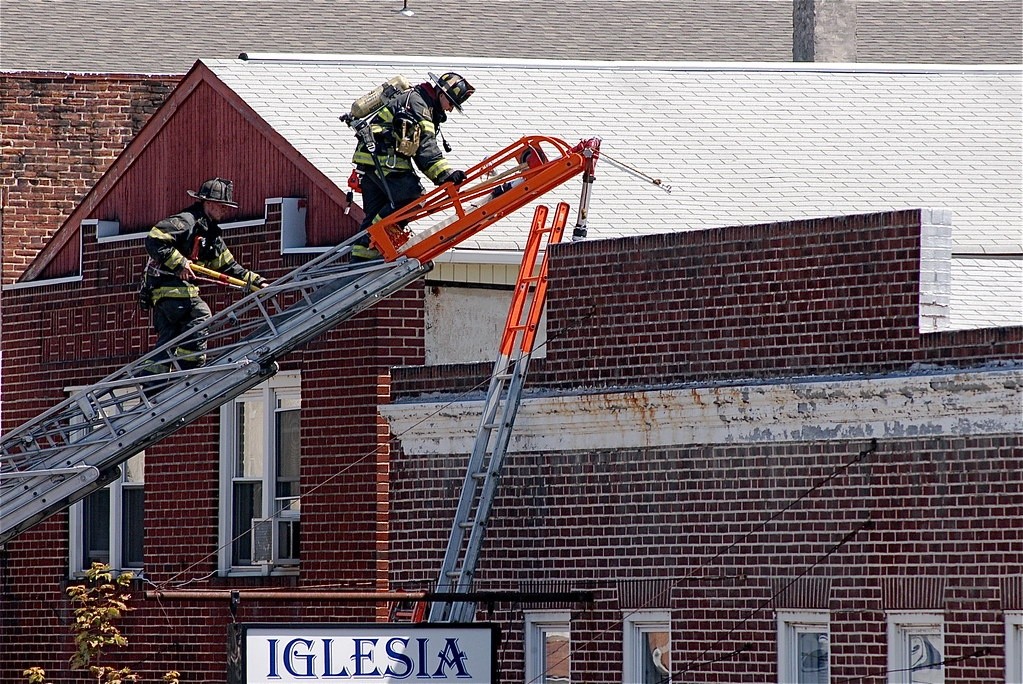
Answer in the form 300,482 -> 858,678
249,518 -> 300,568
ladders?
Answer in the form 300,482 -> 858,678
425,202 -> 571,623
387,587 -> 424,624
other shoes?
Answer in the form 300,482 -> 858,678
348,260 -> 366,270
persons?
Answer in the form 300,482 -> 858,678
139,177 -> 278,404
339,72 -> 477,270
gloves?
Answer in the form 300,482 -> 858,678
444,170 -> 467,184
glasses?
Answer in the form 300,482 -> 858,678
443,94 -> 454,106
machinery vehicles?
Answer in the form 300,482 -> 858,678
0,134 -> 669,544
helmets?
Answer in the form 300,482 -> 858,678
428,72 -> 475,110
187,178 -> 238,208
